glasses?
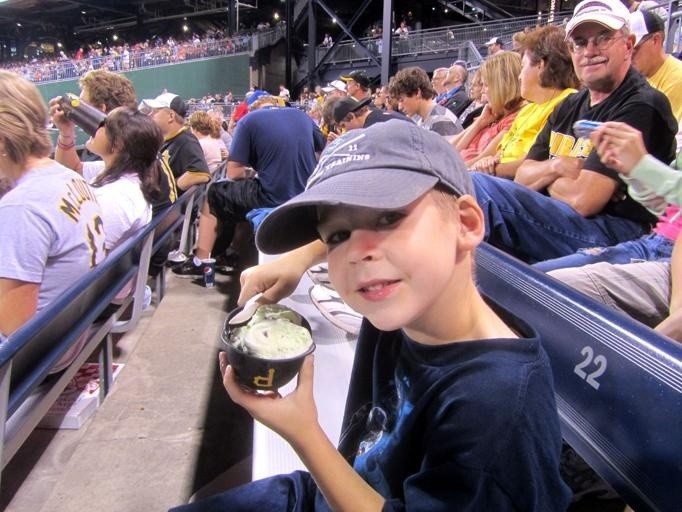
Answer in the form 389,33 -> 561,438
630,35 -> 656,59
568,31 -> 630,51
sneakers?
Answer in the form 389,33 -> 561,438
169,252 -> 214,280
212,253 -> 242,277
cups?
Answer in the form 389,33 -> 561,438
201,259 -> 216,288
58,92 -> 108,136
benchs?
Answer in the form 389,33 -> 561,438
252,241 -> 682,512
0,159 -> 228,471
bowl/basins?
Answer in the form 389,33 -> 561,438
220,304 -> 316,391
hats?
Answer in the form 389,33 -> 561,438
630,8 -> 665,50
246,90 -> 272,107
319,79 -> 348,95
339,68 -> 372,90
253,117 -> 479,255
142,92 -> 187,120
483,34 -> 506,48
333,96 -> 372,125
563,0 -> 633,47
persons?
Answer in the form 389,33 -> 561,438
380,88 -> 394,112
455,49 -> 527,165
322,79 -> 347,99
447,28 -> 455,41
388,66 -> 465,136
173,91 -> 327,278
485,37 -> 502,56
327,98 -> 418,143
323,33 -> 336,65
631,9 -> 681,122
438,61 -> 475,116
340,70 -> 377,109
465,27 -> 582,181
142,93 -> 210,225
432,68 -> 449,102
457,69 -> 486,129
48,95 -> 163,309
396,24 -> 409,54
0,69 -> 106,391
546,229 -> 682,512
168,117 -> 573,512
78,70 -> 178,280
160,84 -> 327,120
530,122 -> 682,274
466,1 -> 678,261
189,112 -> 222,175
0,23 -> 271,84
208,109 -> 232,151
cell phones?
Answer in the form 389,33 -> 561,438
573,120 -> 605,138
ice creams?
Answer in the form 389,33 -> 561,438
230,301 -> 315,362
